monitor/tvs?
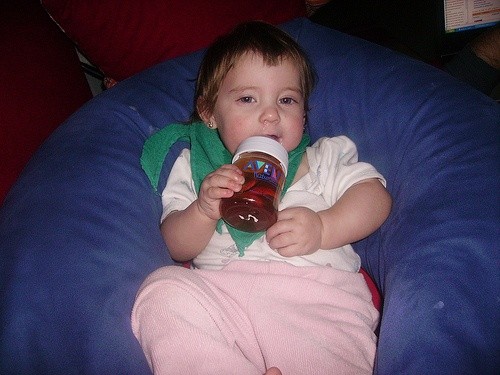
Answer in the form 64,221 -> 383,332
438,0 -> 500,39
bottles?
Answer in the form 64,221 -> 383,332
220,135 -> 288,232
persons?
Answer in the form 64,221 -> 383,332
131,20 -> 392,375
